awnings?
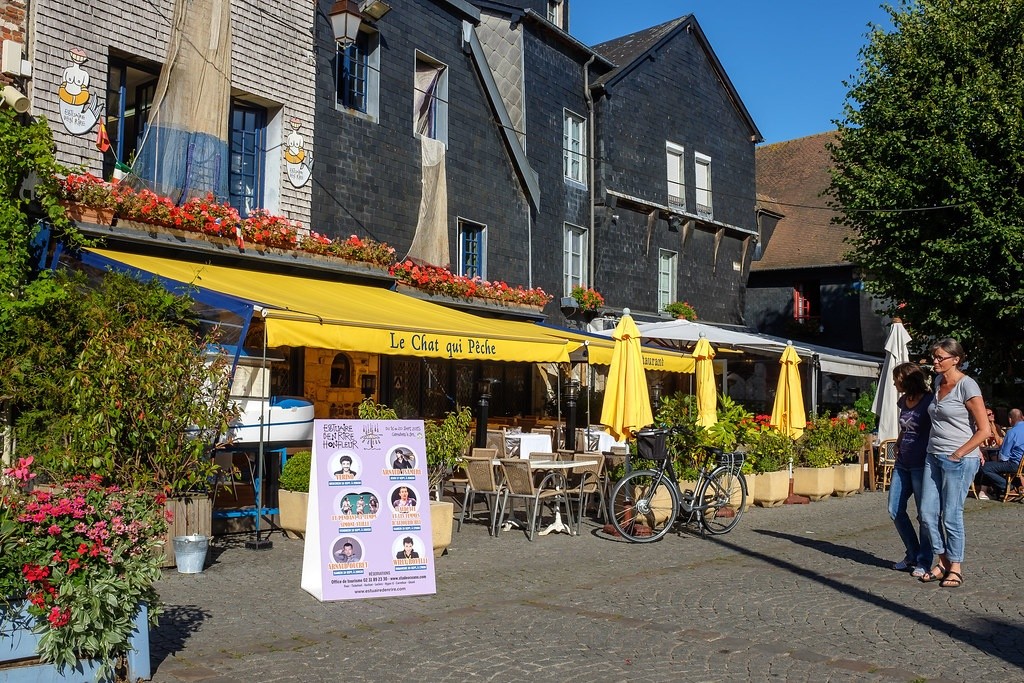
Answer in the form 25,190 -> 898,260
469,313 -> 696,374
82,247 -> 570,363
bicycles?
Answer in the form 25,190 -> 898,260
608,423 -> 749,543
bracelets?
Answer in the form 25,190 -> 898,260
952,452 -> 961,460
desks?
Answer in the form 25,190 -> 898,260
505,433 -> 552,460
530,460 -> 599,536
981,445 -> 1001,462
454,454 -> 551,533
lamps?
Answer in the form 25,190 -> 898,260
668,215 -> 684,228
357,0 -> 393,24
327,0 -> 366,51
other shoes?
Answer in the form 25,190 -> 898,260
1006,496 -> 1015,501
1007,489 -> 1020,495
979,491 -> 993,500
1013,493 -> 1024,501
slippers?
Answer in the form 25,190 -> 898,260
911,563 -> 930,577
893,561 -> 916,570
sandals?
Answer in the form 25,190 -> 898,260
920,564 -> 946,582
941,571 -> 963,588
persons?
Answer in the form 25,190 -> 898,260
922,338 -> 992,586
981,409 -> 1024,501
341,495 -> 376,515
334,456 -> 356,476
887,362 -> 934,577
974,451 -> 995,499
986,409 -> 1002,445
334,543 -> 359,562
393,487 -> 417,507
396,537 -> 419,559
393,449 -> 412,469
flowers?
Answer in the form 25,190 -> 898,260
49,170 -> 554,310
569,283 -> 605,314
736,415 -> 866,432
661,300 -> 698,323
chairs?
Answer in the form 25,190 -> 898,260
875,439 -> 897,492
1003,454 -> 1024,503
437,415 -> 610,541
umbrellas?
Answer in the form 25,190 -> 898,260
769,340 -> 807,478
870,318 -> 913,441
600,308 -> 655,500
692,331 -> 719,435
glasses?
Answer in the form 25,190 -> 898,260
987,412 -> 995,416
931,354 -> 954,361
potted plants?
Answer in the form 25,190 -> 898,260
747,426 -> 796,508
793,405 -> 836,501
358,397 -> 473,558
708,390 -> 755,513
831,405 -> 869,497
278,451 -> 312,540
615,440 -> 680,528
652,390 -> 708,526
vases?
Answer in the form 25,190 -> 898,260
561,297 -> 578,307
659,311 -> 673,319
59,198 -> 117,226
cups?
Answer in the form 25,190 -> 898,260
998,437 -> 1003,446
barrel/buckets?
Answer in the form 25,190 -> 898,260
172,533 -> 214,574
